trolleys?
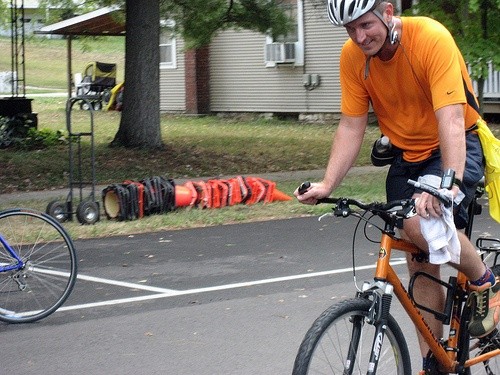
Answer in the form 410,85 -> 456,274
46,96 -> 101,225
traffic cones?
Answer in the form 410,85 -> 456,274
269,182 -> 293,203
168,175 -> 272,210
101,176 -> 176,222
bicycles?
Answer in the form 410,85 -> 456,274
0,207 -> 78,324
291,179 -> 500,375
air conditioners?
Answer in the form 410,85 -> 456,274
264,43 -> 295,63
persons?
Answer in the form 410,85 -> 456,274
291,1 -> 500,374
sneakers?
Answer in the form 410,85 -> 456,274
417,368 -> 449,375
466,276 -> 500,339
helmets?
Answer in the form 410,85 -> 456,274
327,0 -> 375,26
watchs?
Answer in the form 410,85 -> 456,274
439,169 -> 467,193
